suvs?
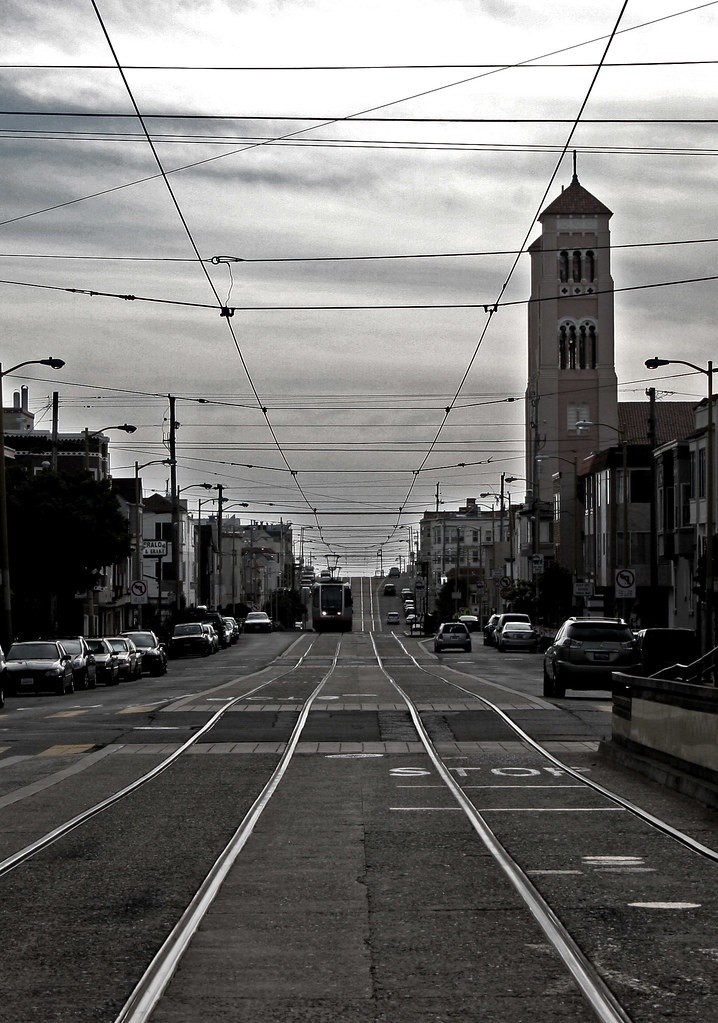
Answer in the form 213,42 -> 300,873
118,629 -> 167,677
434,622 -> 472,653
386,611 -> 400,625
383,583 -> 396,596
482,613 -> 532,647
542,617 -> 644,699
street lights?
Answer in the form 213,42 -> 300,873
479,419 -> 628,619
1,353 -> 250,637
645,356 -> 711,678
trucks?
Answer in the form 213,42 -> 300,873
635,628 -> 703,683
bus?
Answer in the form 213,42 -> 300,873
311,579 -> 354,633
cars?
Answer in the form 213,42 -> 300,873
400,587 -> 418,625
0,640 -> 75,710
85,637 -> 142,686
500,622 -> 541,653
458,615 -> 480,631
389,567 -> 400,577
321,570 -> 330,577
47,636 -> 97,689
172,611 -> 273,658
